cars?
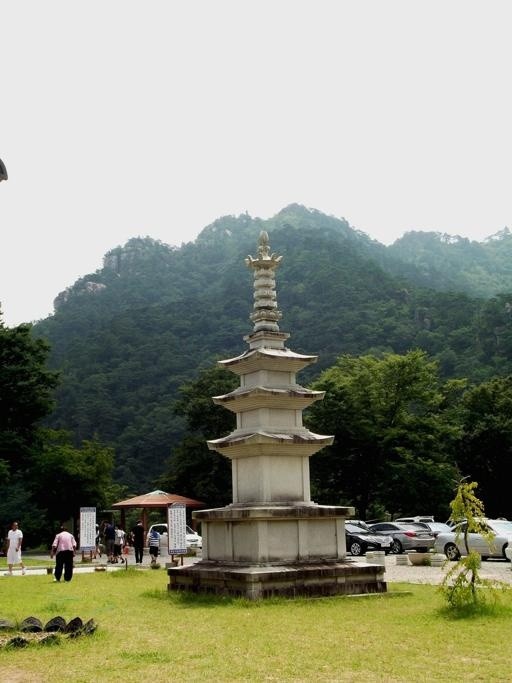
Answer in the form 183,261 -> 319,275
146,524 -> 203,549
345,515 -> 512,562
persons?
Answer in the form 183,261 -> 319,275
95,521 -> 134,564
131,520 -> 147,563
147,528 -> 161,565
51,526 -> 78,582
4,522 -> 26,576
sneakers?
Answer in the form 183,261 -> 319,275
151,560 -> 156,564
4,571 -> 13,575
108,558 -> 124,564
22,566 -> 27,575
136,559 -> 142,563
